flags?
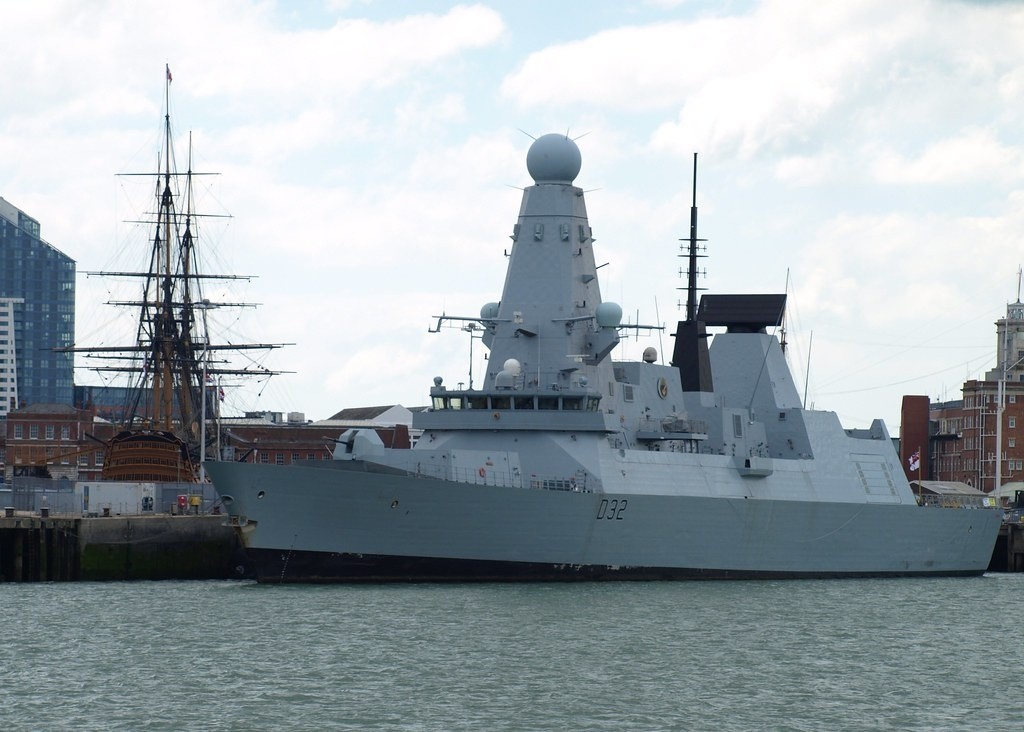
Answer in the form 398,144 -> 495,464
909,452 -> 920,471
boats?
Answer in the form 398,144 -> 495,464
200,129 -> 1009,584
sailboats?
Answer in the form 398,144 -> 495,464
15,63 -> 300,518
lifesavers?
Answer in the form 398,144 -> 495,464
479,468 -> 486,477
493,413 -> 499,420
